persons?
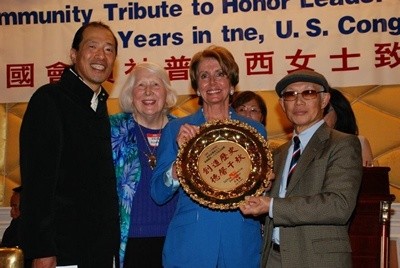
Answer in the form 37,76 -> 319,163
240,70 -> 363,268
108,61 -> 180,268
322,87 -> 359,136
151,46 -> 268,268
0,187 -> 21,248
19,22 -> 120,268
232,91 -> 267,127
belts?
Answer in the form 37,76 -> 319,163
270,242 -> 280,252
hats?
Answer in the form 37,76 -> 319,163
275,70 -> 330,96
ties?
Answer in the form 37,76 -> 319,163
285,135 -> 301,191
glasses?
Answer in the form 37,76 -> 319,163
280,90 -> 326,100
238,106 -> 261,113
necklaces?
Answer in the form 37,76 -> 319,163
135,116 -> 164,168
